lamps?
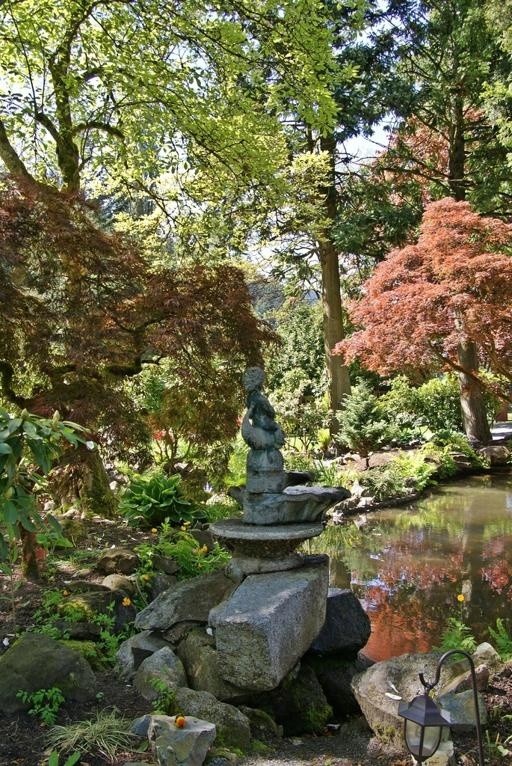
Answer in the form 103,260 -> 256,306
397,651 -> 486,766
